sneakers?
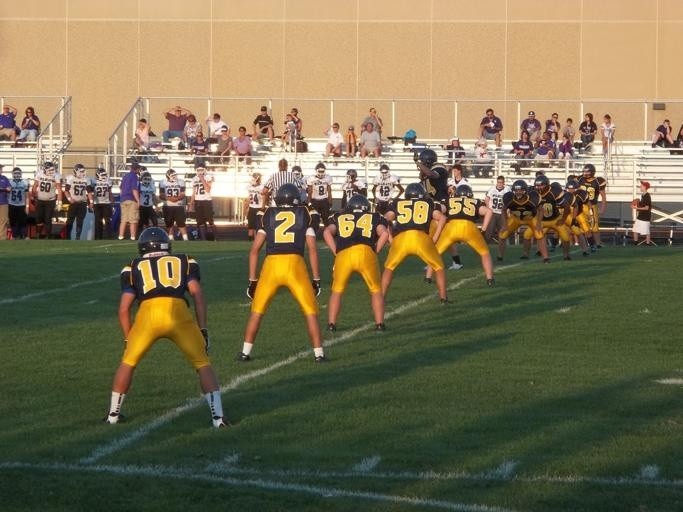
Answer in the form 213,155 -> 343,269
375,324 -> 390,331
237,352 -> 249,362
423,277 -> 432,283
107,411 -> 124,424
520,242 -> 604,264
440,298 -> 448,305
211,416 -> 226,428
448,262 -> 463,270
486,279 -> 494,287
327,322 -> 337,334
313,354 -> 325,362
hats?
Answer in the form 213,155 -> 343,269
640,181 -> 650,189
528,111 -> 536,117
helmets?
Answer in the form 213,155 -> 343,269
405,183 -> 426,200
380,165 -> 390,179
347,195 -> 370,212
141,163 -> 206,188
138,227 -> 172,257
43,162 -> 107,181
511,164 -> 595,201
13,168 -> 21,179
292,163 -> 325,180
420,150 -> 437,168
454,185 -> 474,198
346,170 -> 358,184
274,183 -> 301,207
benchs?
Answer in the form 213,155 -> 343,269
477,224 -> 633,246
0,135 -> 67,191
109,135 -> 682,205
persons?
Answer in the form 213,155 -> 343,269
101,227 -> 230,431
235,184 -> 327,365
0,163 -> 219,242
402,129 -> 417,152
631,180 -> 652,247
425,185 -> 496,288
1,105 -> 41,149
677,125 -> 683,148
244,160 -> 404,241
323,107 -> 383,166
414,149 -> 449,269
133,104 -> 302,172
514,111 -> 598,176
381,184 -> 452,307
601,113 -> 615,171
476,109 -> 502,151
485,164 -> 608,265
441,136 -> 496,178
322,196 -> 389,332
447,163 -> 472,272
652,119 -> 677,155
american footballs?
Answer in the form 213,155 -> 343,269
632,198 -> 640,206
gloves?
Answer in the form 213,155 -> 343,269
247,279 -> 258,301
311,279 -> 321,297
200,328 -> 210,355
